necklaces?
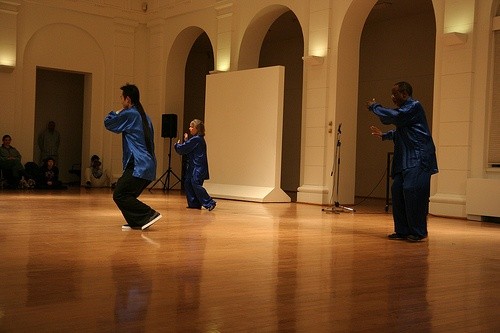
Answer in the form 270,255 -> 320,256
85,155 -> 116,189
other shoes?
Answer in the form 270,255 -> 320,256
122,223 -> 131,228
406,232 -> 427,242
187,206 -> 201,209
141,208 -> 162,230
209,202 -> 216,211
388,233 -> 406,239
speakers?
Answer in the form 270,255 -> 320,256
161,114 -> 178,138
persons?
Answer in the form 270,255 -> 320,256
37,157 -> 59,189
366,81 -> 439,242
173,118 -> 217,211
0,134 -> 27,190
38,121 -> 60,166
103,82 -> 162,230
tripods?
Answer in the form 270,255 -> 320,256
322,124 -> 356,214
149,138 -> 182,194
